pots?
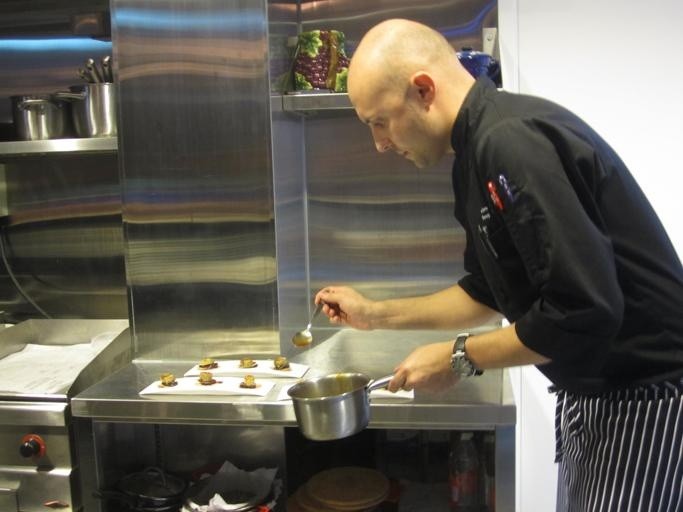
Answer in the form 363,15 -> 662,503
456,45 -> 502,88
88,462 -> 284,511
287,370 -> 401,440
10,80 -> 114,141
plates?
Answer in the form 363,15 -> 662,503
138,356 -> 310,398
292,466 -> 392,510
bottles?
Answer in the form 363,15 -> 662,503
449,432 -> 482,511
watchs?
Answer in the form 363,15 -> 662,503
451,331 -> 485,381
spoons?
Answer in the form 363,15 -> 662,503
290,301 -> 325,351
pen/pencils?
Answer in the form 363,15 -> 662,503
487,174 -> 514,211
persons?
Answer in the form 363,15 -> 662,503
314,19 -> 683,512
